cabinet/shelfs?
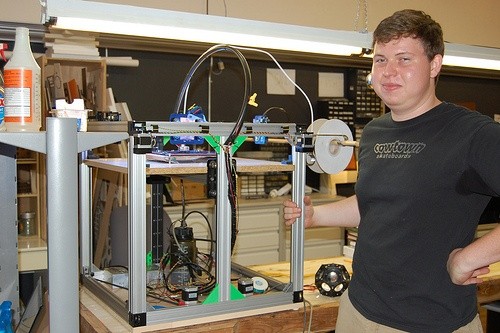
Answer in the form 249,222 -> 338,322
18,148 -> 48,273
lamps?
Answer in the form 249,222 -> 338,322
39,0 -> 500,72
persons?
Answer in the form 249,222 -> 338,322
283,10 -> 500,333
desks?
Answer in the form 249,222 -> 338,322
163,192 -> 345,266
75,255 -> 500,333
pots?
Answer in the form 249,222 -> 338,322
87,111 -> 122,121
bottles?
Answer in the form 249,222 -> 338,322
22,211 -> 34,236
237,278 -> 253,294
0,300 -> 15,333
3,27 -> 42,133
182,285 -> 198,301
170,226 -> 197,270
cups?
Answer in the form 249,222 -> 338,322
48,108 -> 93,132
